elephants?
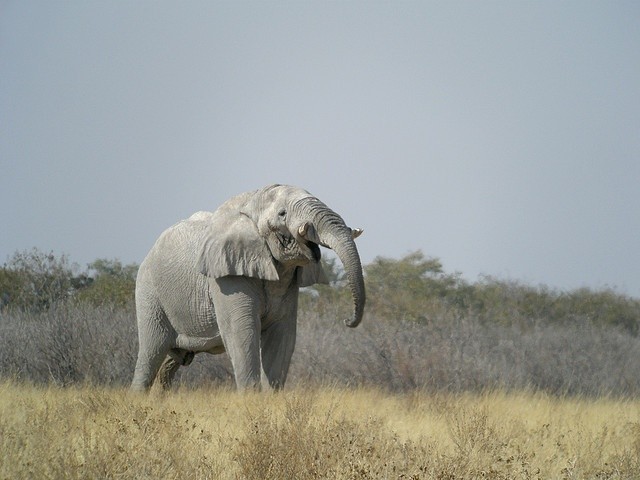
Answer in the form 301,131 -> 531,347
129,184 -> 366,397
